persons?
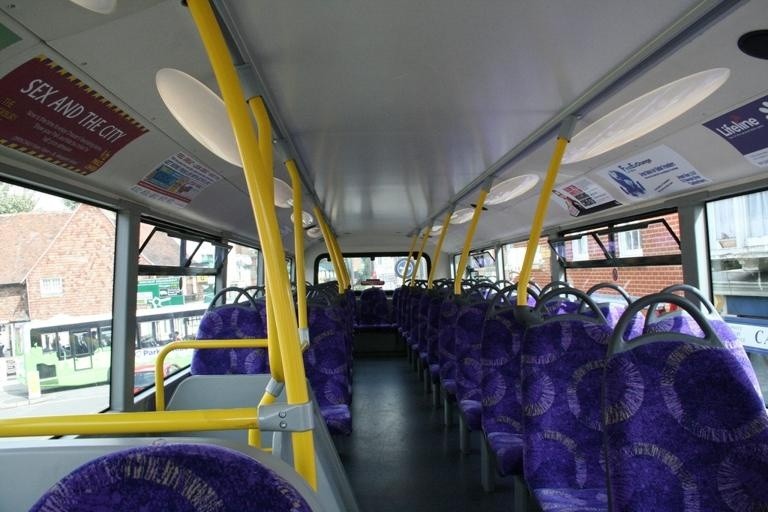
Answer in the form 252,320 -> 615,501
552,190 -> 584,217
175,331 -> 181,341
92,331 -> 97,340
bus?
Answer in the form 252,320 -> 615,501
13,303 -> 211,397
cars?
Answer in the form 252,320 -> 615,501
133,363 -> 181,396
